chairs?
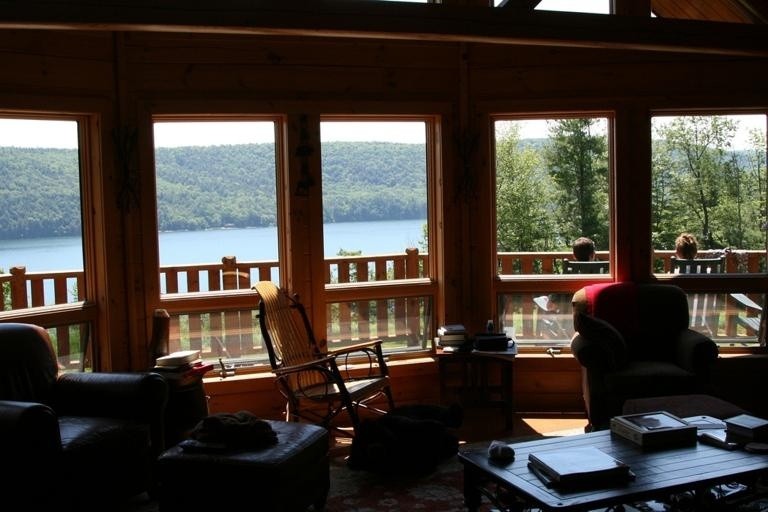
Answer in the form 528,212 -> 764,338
726,294 -> 765,346
540,259 -> 611,342
248,281 -> 395,464
667,253 -> 726,337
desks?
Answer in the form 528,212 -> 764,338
445,416 -> 767,512
427,336 -> 518,435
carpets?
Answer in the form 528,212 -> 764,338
319,429 -> 542,510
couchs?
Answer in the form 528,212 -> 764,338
153,416 -> 330,511
0,322 -> 171,512
565,278 -> 749,432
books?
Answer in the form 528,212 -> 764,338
156,350 -> 201,365
528,462 -> 636,489
434,325 -> 466,348
528,447 -> 632,483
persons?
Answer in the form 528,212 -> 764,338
527,237 -> 606,342
664,234 -> 701,277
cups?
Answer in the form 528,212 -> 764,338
223,364 -> 235,375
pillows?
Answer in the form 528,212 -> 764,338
572,308 -> 631,368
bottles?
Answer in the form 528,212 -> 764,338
485,318 -> 495,335
